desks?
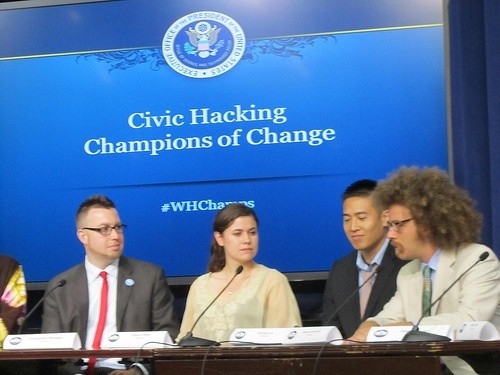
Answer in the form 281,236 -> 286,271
0,341 -> 500,375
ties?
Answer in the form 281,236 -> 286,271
422,265 -> 433,317
87,271 -> 108,375
360,264 -> 378,322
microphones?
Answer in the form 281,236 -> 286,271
17,278 -> 66,334
403,251 -> 490,342
322,264 -> 386,326
177,265 -> 244,347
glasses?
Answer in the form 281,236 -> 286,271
81,224 -> 127,236
386,217 -> 415,232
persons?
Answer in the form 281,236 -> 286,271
175,203 -> 302,343
40,195 -> 181,375
348,165 -> 500,342
0,254 -> 28,348
323,179 -> 413,340
